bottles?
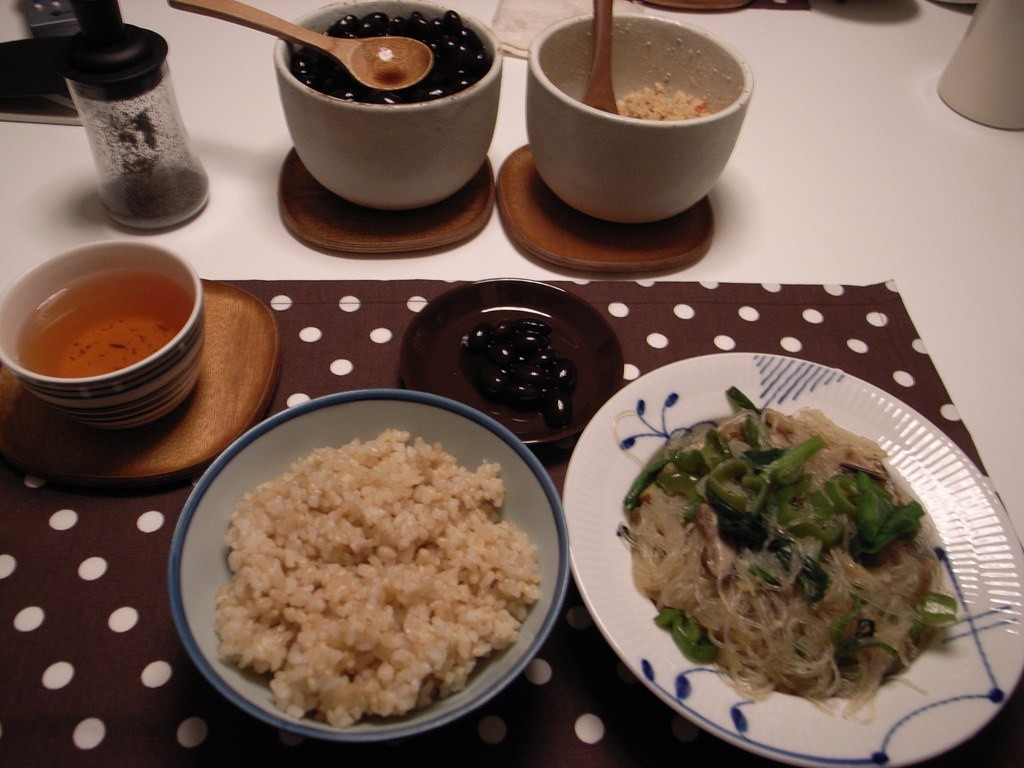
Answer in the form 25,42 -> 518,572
59,0 -> 207,229
937,0 -> 1024,130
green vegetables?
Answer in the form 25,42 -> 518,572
623,385 -> 960,682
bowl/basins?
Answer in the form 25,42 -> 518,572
273,0 -> 503,210
525,13 -> 753,224
0,241 -> 206,428
166,388 -> 573,740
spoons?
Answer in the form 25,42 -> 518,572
165,0 -> 433,90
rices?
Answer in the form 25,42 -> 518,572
214,426 -> 543,729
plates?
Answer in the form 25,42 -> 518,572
562,353 -> 1024,768
399,278 -> 624,444
